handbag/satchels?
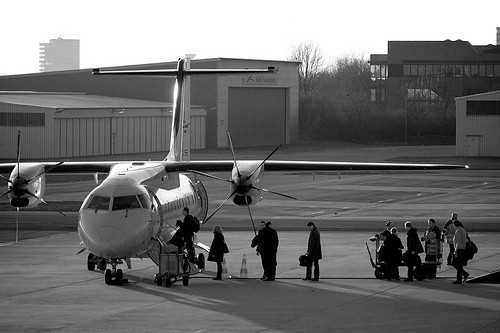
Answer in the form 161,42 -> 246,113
299,253 -> 309,266
466,241 -> 478,260
224,243 -> 229,253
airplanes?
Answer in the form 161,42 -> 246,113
0,52 -> 470,289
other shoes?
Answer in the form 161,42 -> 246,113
313,277 -> 319,281
464,273 -> 469,282
303,277 -> 311,280
213,275 -> 221,280
404,278 -> 413,282
452,281 -> 462,284
396,278 -> 400,280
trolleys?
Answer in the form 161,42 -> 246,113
368,233 -> 444,279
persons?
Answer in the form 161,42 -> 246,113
301,221 -> 322,281
451,220 -> 469,284
375,222 -> 404,281
421,218 -> 441,279
441,212 -> 465,265
207,225 -> 225,281
181,207 -> 194,264
256,221 -> 279,281
404,222 -> 424,282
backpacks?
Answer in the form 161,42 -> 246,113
192,216 -> 200,233
